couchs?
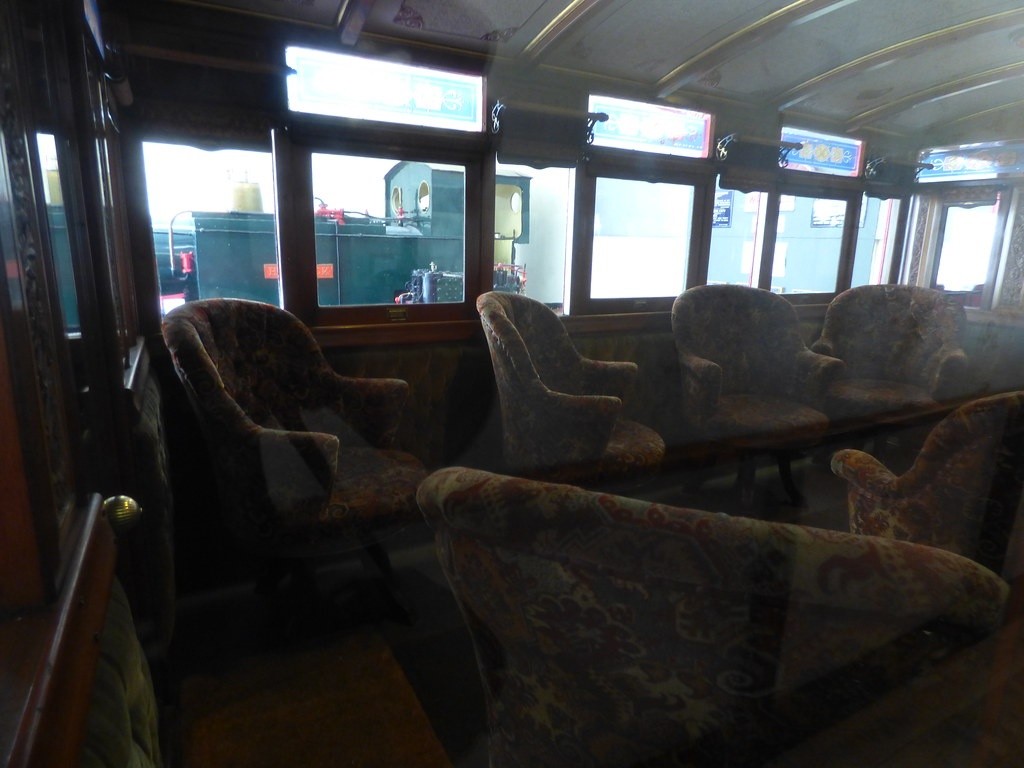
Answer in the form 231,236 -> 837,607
163,298 -> 430,626
831,390 -> 1024,584
809,285 -> 969,425
414,467 -> 1010,768
476,290 -> 666,486
670,284 -> 845,451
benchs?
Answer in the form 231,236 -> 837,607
4,491 -> 455,768
